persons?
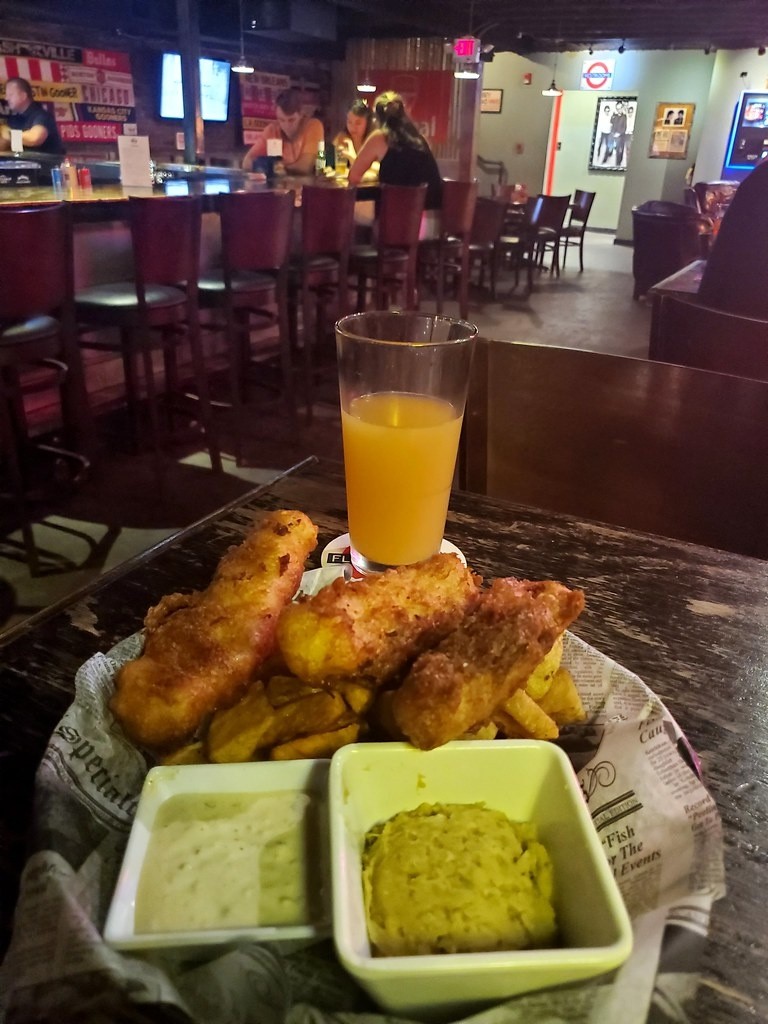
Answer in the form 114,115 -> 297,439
347,91 -> 442,305
664,110 -> 674,124
674,110 -> 684,124
242,89 -> 324,176
0,77 -> 66,154
598,101 -> 635,167
333,98 -> 380,173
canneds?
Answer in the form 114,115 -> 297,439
50,168 -> 63,189
77,168 -> 91,189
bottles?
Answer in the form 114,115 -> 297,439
315,141 -> 327,173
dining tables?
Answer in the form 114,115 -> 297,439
1,457 -> 768,1023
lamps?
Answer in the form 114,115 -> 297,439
231,0 -> 254,73
357,0 -> 376,92
453,0 -> 480,80
619,38 -> 627,53
541,0 -> 565,96
758,44 -> 766,55
705,39 -> 717,55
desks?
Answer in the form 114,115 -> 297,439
0,150 -> 379,451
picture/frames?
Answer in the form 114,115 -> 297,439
648,102 -> 696,160
588,96 -> 638,171
481,88 -> 503,114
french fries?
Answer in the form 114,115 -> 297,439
159,632 -> 584,765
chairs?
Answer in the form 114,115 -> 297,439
0,177 -> 768,574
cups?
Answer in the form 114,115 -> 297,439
336,310 -> 480,574
334,145 -> 349,175
60,164 -> 78,188
78,169 -> 91,188
102,759 -> 331,956
327,739 -> 635,1020
51,169 -> 62,189
11,130 -> 23,152
